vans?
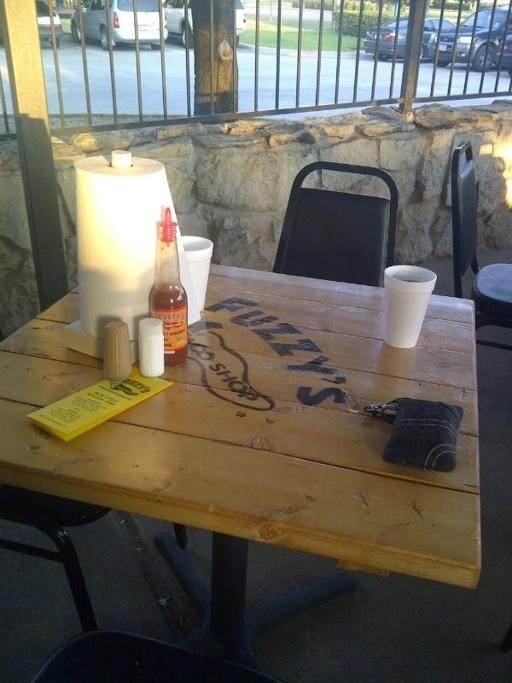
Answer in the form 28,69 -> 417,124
72,1 -> 168,49
162,0 -> 247,47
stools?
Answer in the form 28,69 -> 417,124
28,626 -> 285,681
0,482 -> 191,628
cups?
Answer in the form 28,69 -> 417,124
179,233 -> 214,310
380,262 -> 436,349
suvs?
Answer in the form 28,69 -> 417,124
427,3 -> 511,70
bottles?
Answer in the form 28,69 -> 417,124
149,206 -> 188,364
139,318 -> 165,374
102,316 -> 133,379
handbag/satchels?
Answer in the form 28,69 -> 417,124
364,397 -> 463,473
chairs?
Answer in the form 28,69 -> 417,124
274,160 -> 401,287
452,140 -> 512,358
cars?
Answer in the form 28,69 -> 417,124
495,30 -> 512,76
36,1 -> 64,47
363,17 -> 457,61
